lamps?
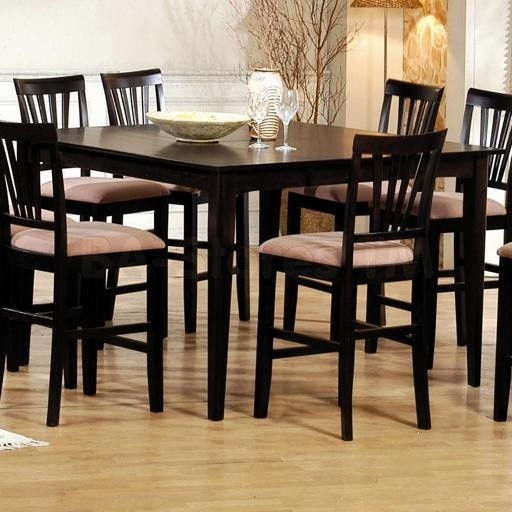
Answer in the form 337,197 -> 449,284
350,0 -> 426,132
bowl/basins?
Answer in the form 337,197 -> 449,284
146,109 -> 251,144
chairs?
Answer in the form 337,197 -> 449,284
101,68 -> 251,333
14,74 -> 170,340
494,243 -> 511,420
2,122 -> 168,426
368,87 -> 512,346
280,78 -> 445,354
253,128 -> 449,441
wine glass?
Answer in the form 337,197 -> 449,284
275,88 -> 300,153
247,82 -> 269,149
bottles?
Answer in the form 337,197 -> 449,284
248,69 -> 281,142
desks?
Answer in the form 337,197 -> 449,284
57,122 -> 506,421
